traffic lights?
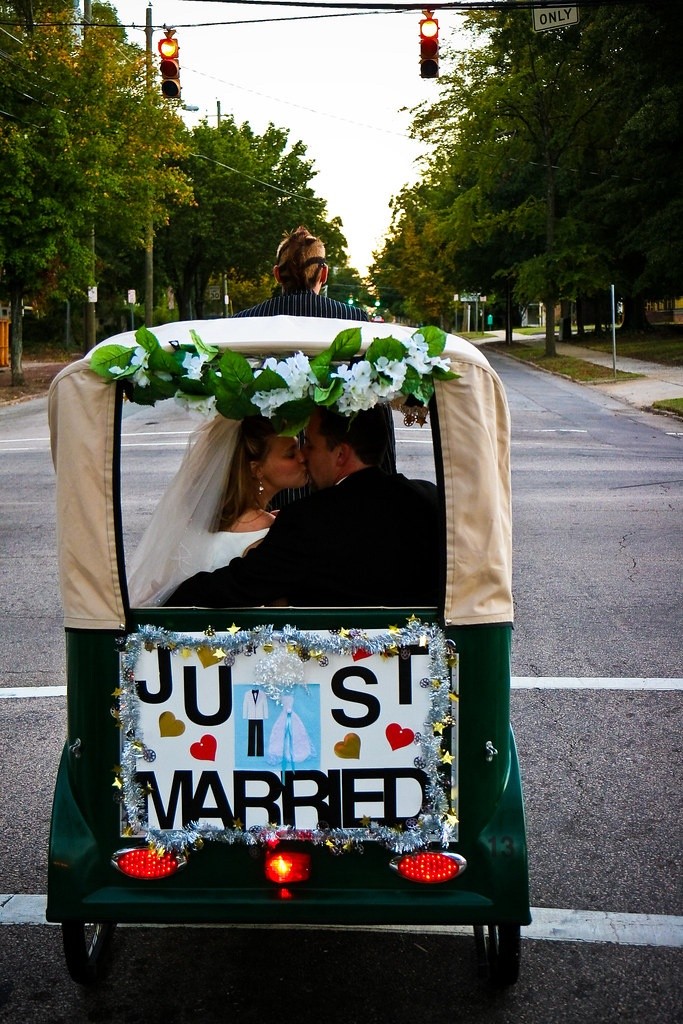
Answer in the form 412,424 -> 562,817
159,39 -> 181,98
420,19 -> 439,78
375,296 -> 381,306
348,293 -> 354,305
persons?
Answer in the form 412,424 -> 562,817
231,227 -> 396,511
164,404 -> 439,607
127,415 -> 310,607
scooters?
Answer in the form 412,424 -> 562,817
45,315 -> 532,991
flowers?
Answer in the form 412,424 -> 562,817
88,324 -> 464,438
